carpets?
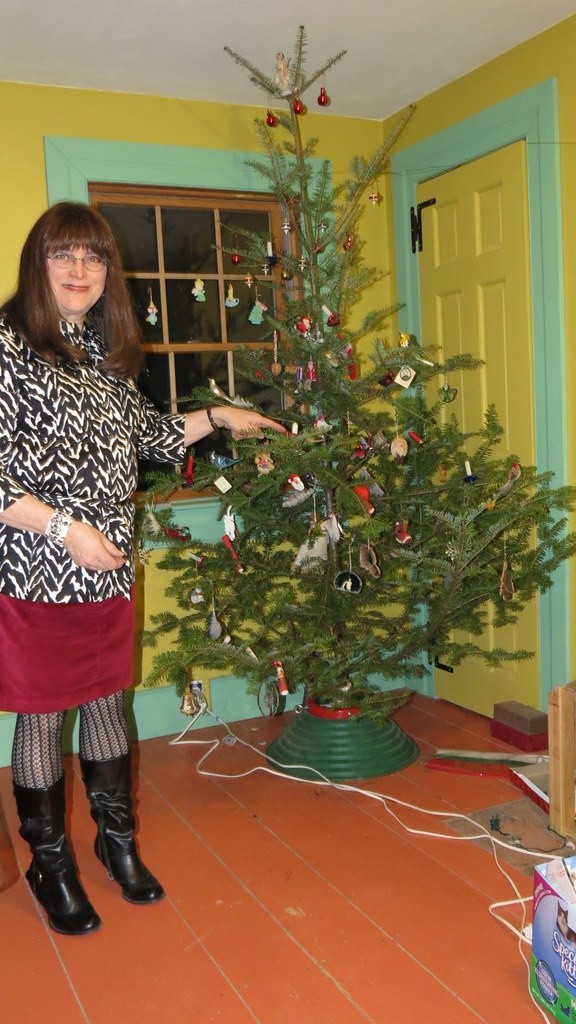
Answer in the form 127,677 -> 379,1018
440,795 -> 576,878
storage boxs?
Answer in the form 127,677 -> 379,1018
508,762 -> 550,816
530,856 -> 576,1024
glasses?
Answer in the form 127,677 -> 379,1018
46,251 -> 107,273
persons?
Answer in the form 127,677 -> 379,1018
0,202 -> 287,934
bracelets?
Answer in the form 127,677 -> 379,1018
45,511 -> 73,548
207,407 -> 217,430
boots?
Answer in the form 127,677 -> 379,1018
12,768 -> 101,935
78,748 -> 164,903
